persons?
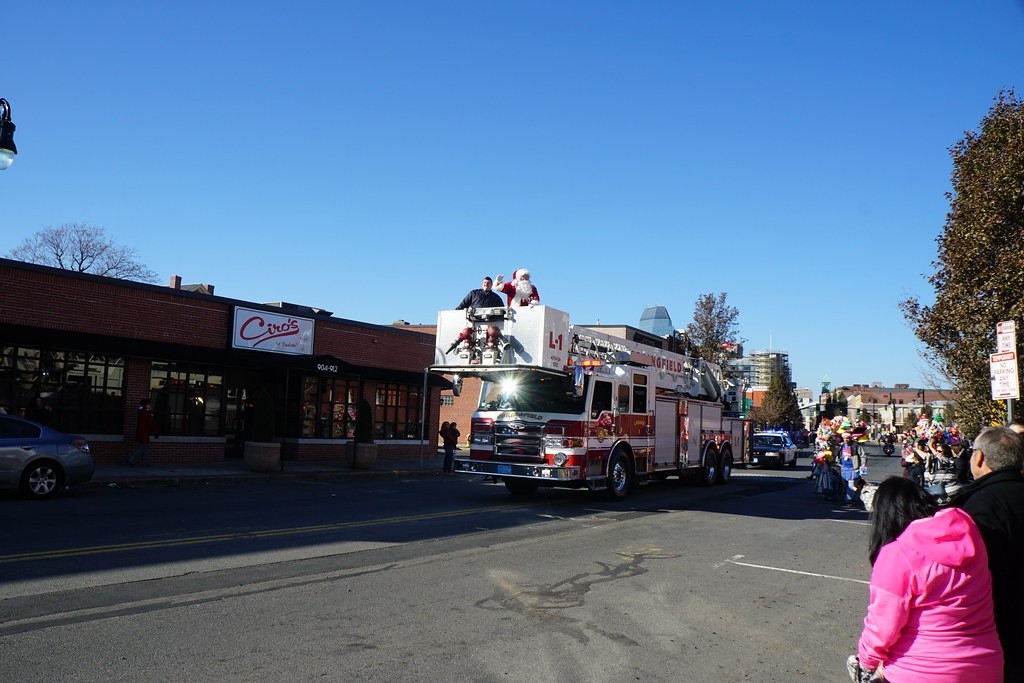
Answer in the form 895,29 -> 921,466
907,452 -> 926,486
857,475 -> 1004,683
455,276 -> 504,310
833,431 -> 867,509
901,458 -> 912,478
126,398 -> 160,469
902,426 -> 975,484
438,421 -> 460,477
941,425 -> 1024,683
807,461 -> 814,479
491,268 -> 539,307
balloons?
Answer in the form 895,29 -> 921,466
813,415 -> 855,464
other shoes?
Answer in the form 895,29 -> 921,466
127,459 -> 134,468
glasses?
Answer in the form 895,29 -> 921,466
936,450 -> 944,453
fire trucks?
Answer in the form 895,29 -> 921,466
423,295 -> 753,497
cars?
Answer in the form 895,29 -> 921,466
750,431 -> 798,469
0,410 -> 94,498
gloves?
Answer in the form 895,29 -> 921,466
496,274 -> 505,283
528,303 -> 536,308
847,653 -> 875,683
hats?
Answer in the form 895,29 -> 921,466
511,268 -> 530,286
